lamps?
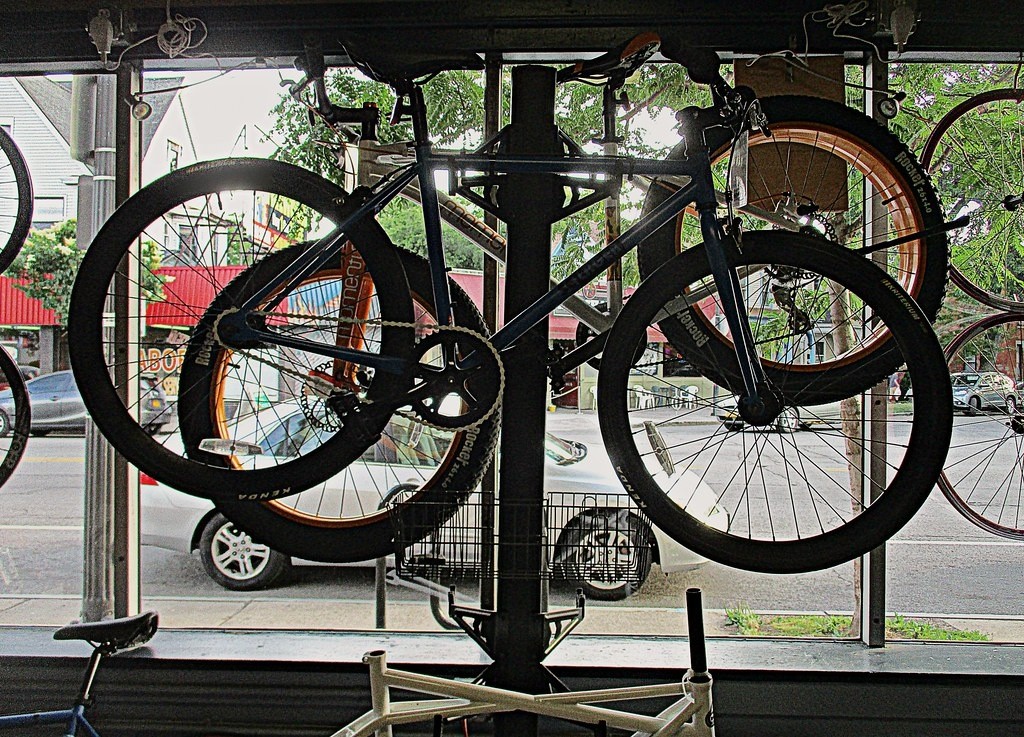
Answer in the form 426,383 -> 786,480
124,93 -> 153,122
875,91 -> 908,119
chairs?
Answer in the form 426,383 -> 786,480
409,422 -> 441,464
589,384 -> 699,411
393,426 -> 419,465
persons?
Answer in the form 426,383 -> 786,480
889,372 -> 899,403
898,373 -> 910,401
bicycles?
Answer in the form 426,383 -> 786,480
0,23 -> 1023,577
1,609 -> 160,737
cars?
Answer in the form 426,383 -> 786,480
139,395 -> 730,602
1015,382 -> 1024,410
0,368 -> 172,437
950,372 -> 1019,416
1,366 -> 42,392
715,394 -> 863,434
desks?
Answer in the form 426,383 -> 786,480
661,387 -> 670,406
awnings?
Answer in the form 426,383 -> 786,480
413,273 -> 668,343
